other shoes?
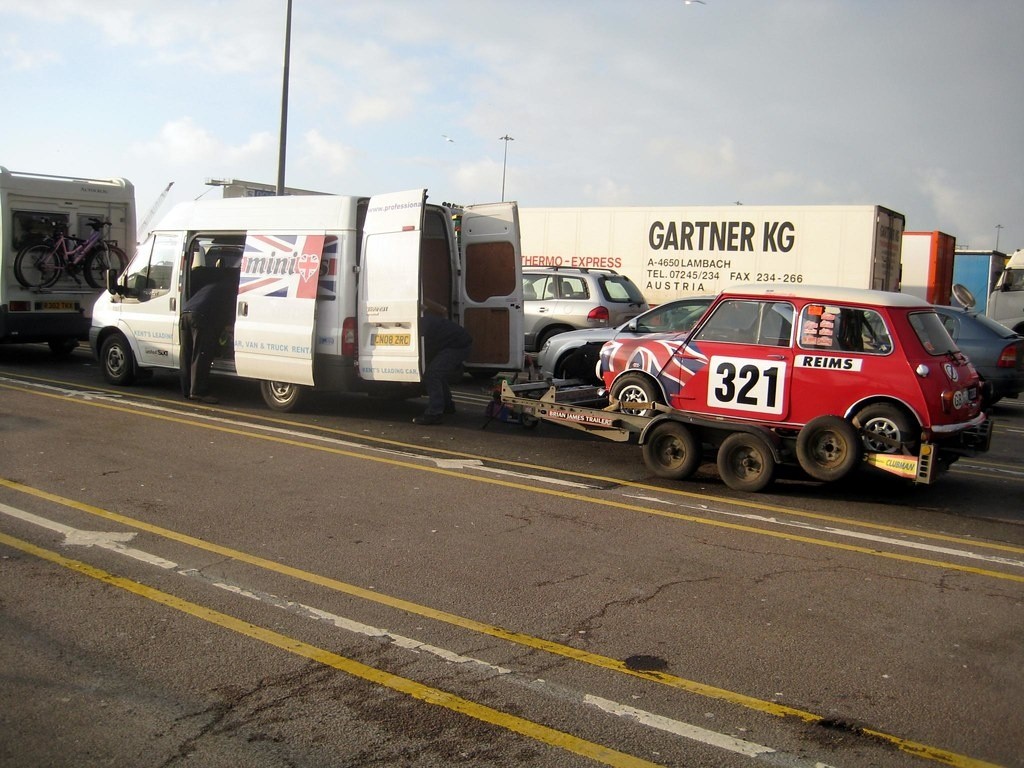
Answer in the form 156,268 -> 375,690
412,414 -> 441,425
443,402 -> 456,413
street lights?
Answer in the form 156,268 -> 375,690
498,133 -> 517,201
992,221 -> 1004,253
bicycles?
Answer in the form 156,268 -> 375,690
14,212 -> 129,291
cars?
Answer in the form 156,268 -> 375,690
836,299 -> 1024,413
596,280 -> 991,463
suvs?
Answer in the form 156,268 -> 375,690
511,264 -> 650,359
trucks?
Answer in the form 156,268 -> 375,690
986,248 -> 1024,353
435,195 -> 907,322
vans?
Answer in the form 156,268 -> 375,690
0,164 -> 137,362
86,185 -> 529,424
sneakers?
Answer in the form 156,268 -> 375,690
184,393 -> 219,404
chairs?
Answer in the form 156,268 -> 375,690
523,281 -> 574,298
731,314 -> 885,354
190,266 -> 241,298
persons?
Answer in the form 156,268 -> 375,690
412,314 -> 473,425
179,280 -> 239,404
